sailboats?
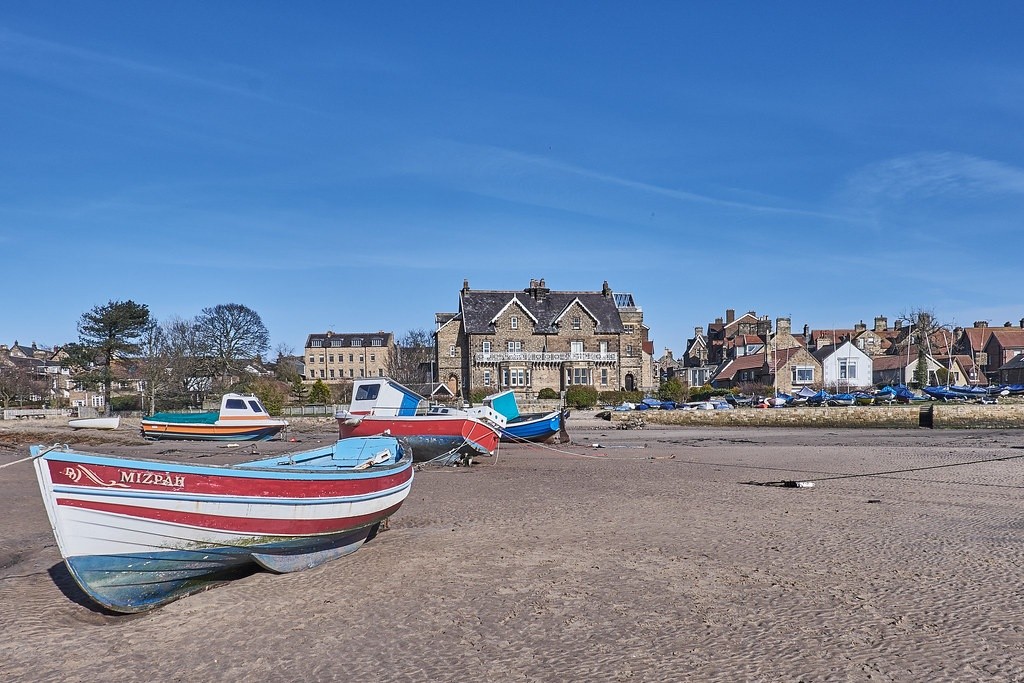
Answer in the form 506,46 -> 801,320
769,319 -> 1023,407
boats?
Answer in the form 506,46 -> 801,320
482,392 -> 568,441
140,391 -> 288,443
425,402 -> 506,434
609,391 -> 765,411
66,416 -> 120,432
331,378 -> 503,467
27,428 -> 416,616
